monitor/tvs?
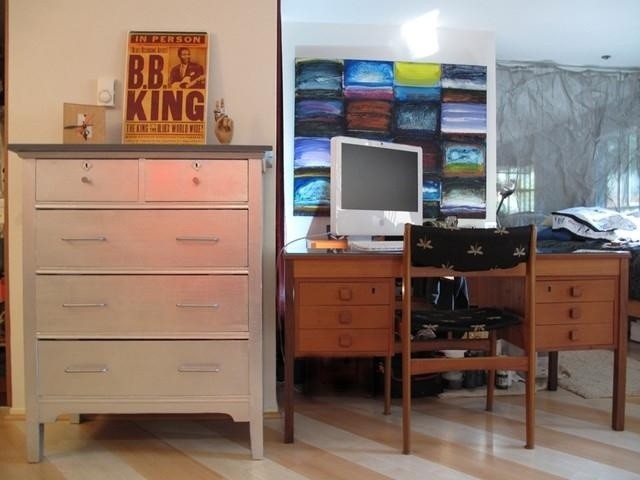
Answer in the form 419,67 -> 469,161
330,134 -> 424,237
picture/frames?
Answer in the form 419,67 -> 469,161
61,102 -> 109,146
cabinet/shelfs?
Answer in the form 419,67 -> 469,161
3,138 -> 275,466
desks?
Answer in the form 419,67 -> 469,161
277,244 -> 634,447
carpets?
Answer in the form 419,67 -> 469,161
536,345 -> 640,401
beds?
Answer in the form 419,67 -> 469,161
541,206 -> 640,320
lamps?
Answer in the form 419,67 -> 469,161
497,178 -> 515,221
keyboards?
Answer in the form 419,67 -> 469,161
349,239 -> 404,252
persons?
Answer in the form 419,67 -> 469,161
215,98 -> 234,144
167,46 -> 205,90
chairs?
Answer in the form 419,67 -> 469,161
383,221 -> 541,452
